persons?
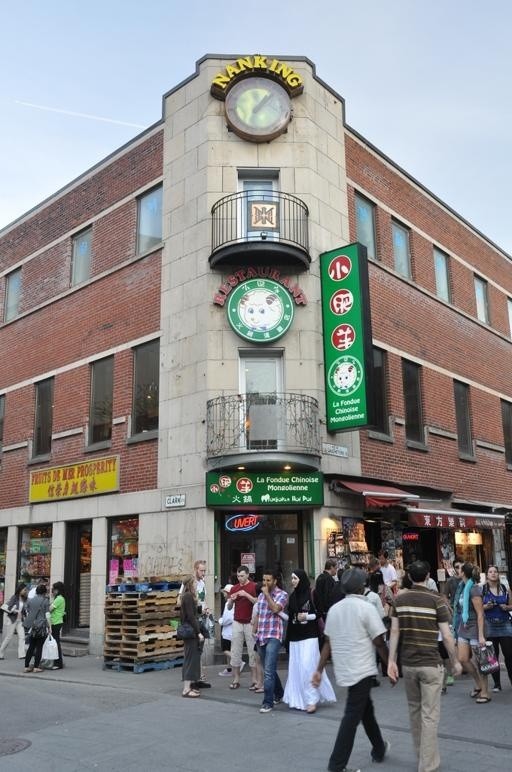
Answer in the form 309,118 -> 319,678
216,583 -> 248,679
249,571 -> 291,714
180,573 -> 206,697
226,565 -> 259,689
284,566 -> 322,714
46,581 -> 67,670
176,559 -> 215,688
386,559 -> 465,772
314,560 -> 511,705
23,584 -> 53,672
0,583 -> 31,660
308,566 -> 399,772
251,588 -> 272,695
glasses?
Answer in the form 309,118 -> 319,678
456,566 -> 463,568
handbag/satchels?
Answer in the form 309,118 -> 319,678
470,640 -> 498,674
30,620 -> 46,637
176,623 -> 198,640
0,603 -> 16,613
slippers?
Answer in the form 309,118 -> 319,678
182,689 -> 200,698
218,662 -> 264,693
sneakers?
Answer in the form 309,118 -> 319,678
190,681 -> 210,689
24,667 -> 32,672
307,705 -> 316,713
33,668 -> 42,673
273,691 -> 284,704
471,688 -> 481,698
476,696 -> 491,703
372,741 -> 390,761
493,688 -> 502,692
18,657 -> 25,660
52,666 -> 62,670
260,706 -> 272,712
0,658 -> 5,660
343,769 -> 360,772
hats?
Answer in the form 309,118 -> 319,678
220,584 -> 233,593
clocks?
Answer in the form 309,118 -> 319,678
223,75 -> 295,143
